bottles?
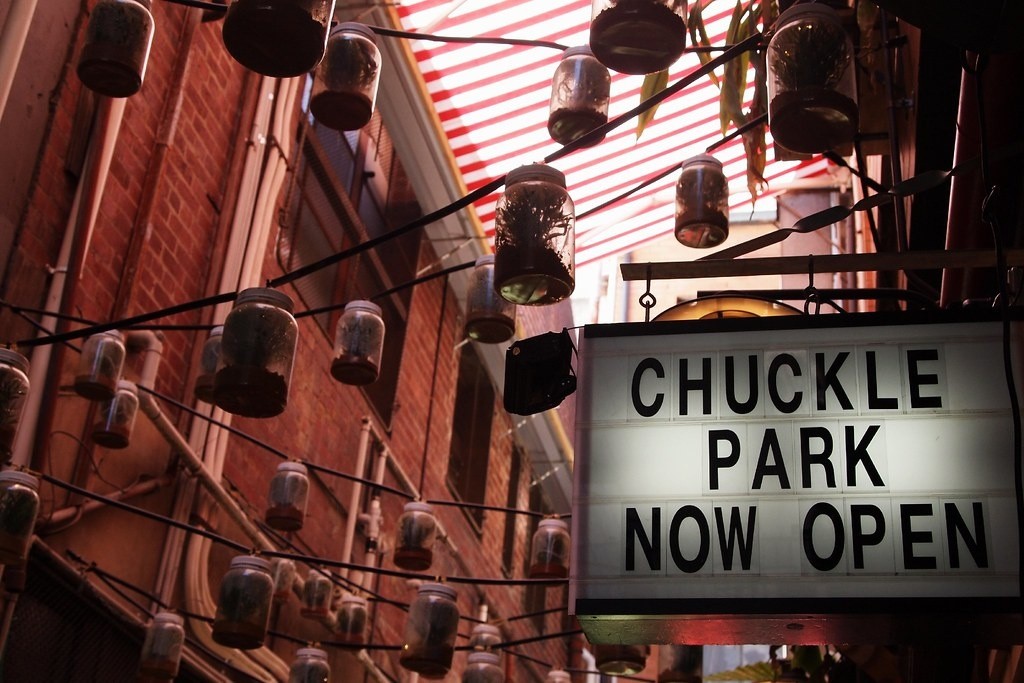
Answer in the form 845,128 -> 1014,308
546,47 -> 612,150
399,584 -> 461,675
74,328 -> 139,450
330,300 -> 384,387
75,0 -> 154,98
766,1 -> 860,154
265,461 -> 311,530
195,284 -> 299,417
0,348 -> 41,598
528,518 -> 570,586
459,623 -> 505,683
392,503 -> 437,571
464,165 -> 575,345
223,0 -> 335,78
135,613 -> 185,676
590,0 -> 689,76
593,642 -> 645,677
675,155 -> 729,247
211,556 -> 369,683
310,21 -> 382,131
